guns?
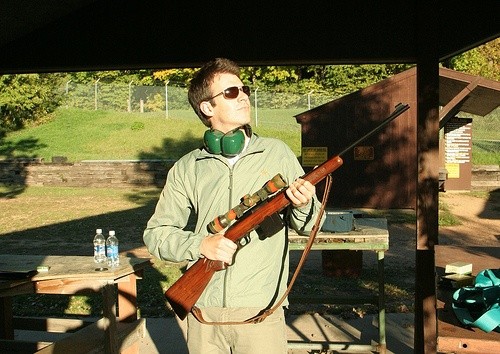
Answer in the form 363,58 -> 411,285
165,104 -> 411,320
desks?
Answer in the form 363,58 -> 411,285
287,218 -> 389,354
0,256 -> 154,354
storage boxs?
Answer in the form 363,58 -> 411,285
441,274 -> 475,290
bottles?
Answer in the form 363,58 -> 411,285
106,230 -> 119,268
93,229 -> 105,263
